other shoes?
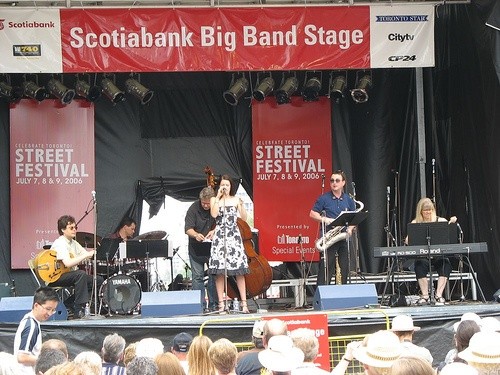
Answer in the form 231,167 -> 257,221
72,309 -> 87,319
201,301 -> 250,315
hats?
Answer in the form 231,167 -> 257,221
457,332 -> 500,363
453,313 -> 482,332
351,330 -> 402,368
252,321 -> 269,338
171,332 -> 193,352
258,335 -> 305,372
481,317 -> 500,332
391,315 -> 421,332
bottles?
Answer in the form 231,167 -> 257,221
233,297 -> 239,315
10,280 -> 16,297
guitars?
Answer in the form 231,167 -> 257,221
34,249 -> 116,283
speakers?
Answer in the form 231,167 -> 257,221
0,297 -> 70,321
141,289 -> 203,318
312,282 -> 379,310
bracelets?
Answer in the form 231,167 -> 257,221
193,234 -> 198,238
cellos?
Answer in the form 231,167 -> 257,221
204,166 -> 273,300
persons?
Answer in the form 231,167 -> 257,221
0,286 -> 500,375
309,170 -> 355,290
209,174 -> 251,314
184,187 -> 219,314
404,197 -> 458,304
110,217 -> 136,240
47,215 -> 107,320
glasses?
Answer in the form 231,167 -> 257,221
37,302 -> 56,314
330,178 -> 343,183
67,226 -> 78,230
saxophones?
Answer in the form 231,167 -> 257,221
315,192 -> 365,250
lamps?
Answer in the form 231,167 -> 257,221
252,71 -> 276,102
46,73 -> 76,105
73,73 -> 102,102
273,71 -> 299,105
301,71 -> 324,103
100,73 -> 126,104
121,73 -> 154,104
0,74 -> 23,107
350,68 -> 375,104
223,72 -> 248,107
326,69 -> 349,104
21,74 -> 49,104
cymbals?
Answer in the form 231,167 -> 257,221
72,232 -> 103,248
133,231 -> 167,240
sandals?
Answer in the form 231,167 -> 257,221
414,294 -> 445,305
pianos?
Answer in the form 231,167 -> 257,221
374,241 -> 489,257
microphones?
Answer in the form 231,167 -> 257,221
351,181 -> 356,197
223,187 -> 227,194
10,279 -> 17,296
298,234 -> 301,244
91,191 -> 96,202
321,173 -> 325,187
390,169 -> 398,174
387,186 -> 391,194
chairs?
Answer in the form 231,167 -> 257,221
28,258 -> 71,305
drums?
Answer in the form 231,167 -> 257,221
97,261 -> 150,314
